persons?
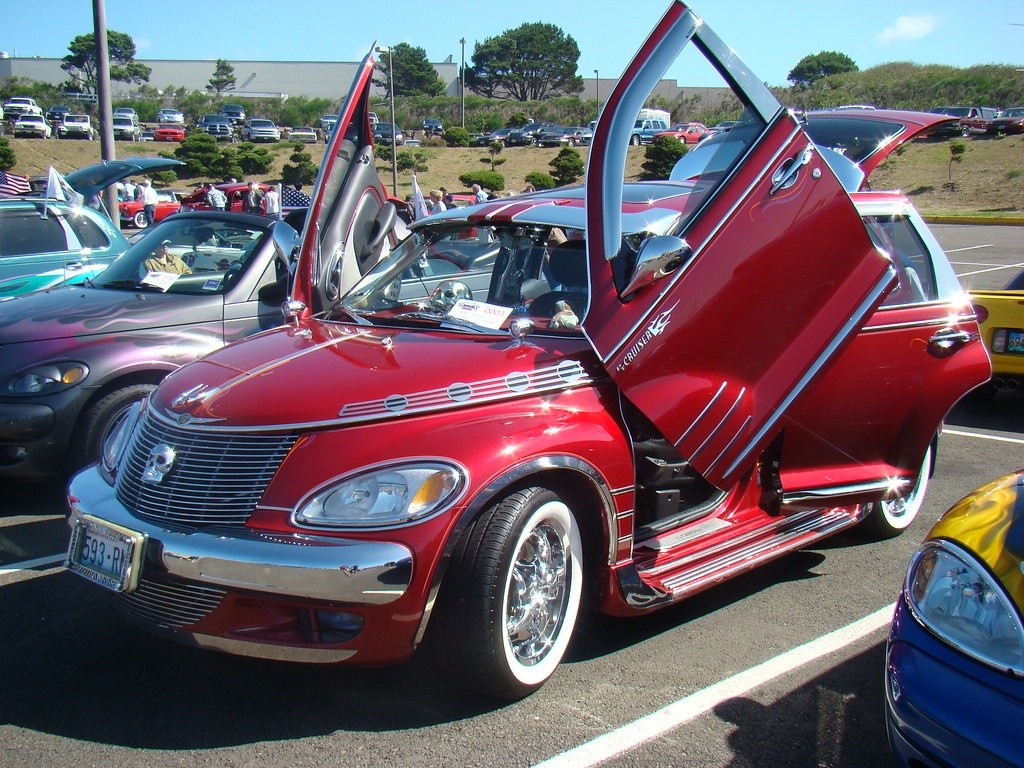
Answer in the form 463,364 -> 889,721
242,182 -> 279,219
547,227 -> 568,255
141,180 -> 159,226
193,178 -> 240,196
144,240 -> 193,275
88,179 -> 142,210
24,174 -> 44,191
430,184 -> 537,215
207,184 -> 227,211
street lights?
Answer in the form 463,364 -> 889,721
593,69 -> 599,119
459,37 -> 466,128
374,45 -> 397,197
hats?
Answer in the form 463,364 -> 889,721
162,240 -> 171,245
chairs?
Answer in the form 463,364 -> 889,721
527,240 -> 590,322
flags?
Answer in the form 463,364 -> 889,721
0,171 -> 32,195
279,185 -> 311,207
412,175 -> 428,221
47,165 -> 84,204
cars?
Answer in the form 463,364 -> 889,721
155,109 -> 184,124
240,119 -> 280,142
112,108 -> 142,142
193,105 -> 248,141
0,159 -> 188,302
883,270 -> 1023,768
404,107 -> 744,258
140,124 -> 186,142
3,98 -> 52,139
23,175 -> 269,228
62,0 -> 992,702
926,107 -> 1024,137
45,107 -> 74,124
56,115 -> 93,140
0,212 -> 557,479
320,111 -> 405,146
287,127 -> 318,145
